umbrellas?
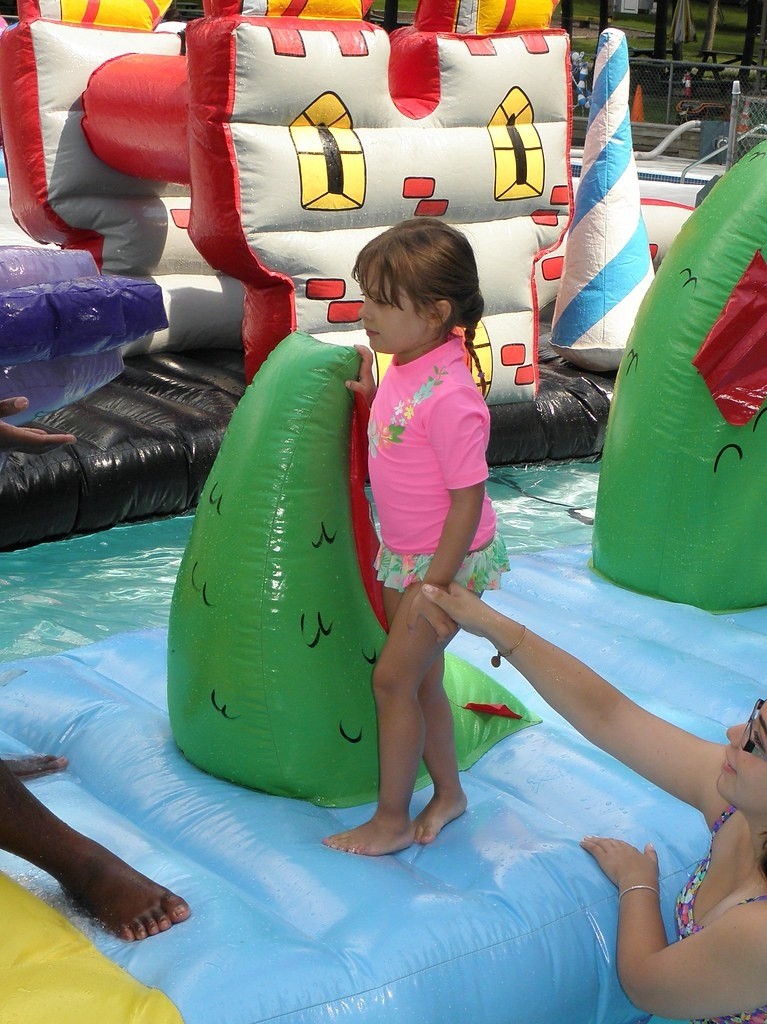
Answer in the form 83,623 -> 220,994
669,0 -> 698,61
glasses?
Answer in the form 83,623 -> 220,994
740,698 -> 767,761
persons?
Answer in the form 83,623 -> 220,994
1,395 -> 189,943
420,583 -> 767,1024
674,67 -> 708,125
321,220 -> 510,855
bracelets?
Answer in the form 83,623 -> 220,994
491,625 -> 526,668
619,885 -> 660,898
